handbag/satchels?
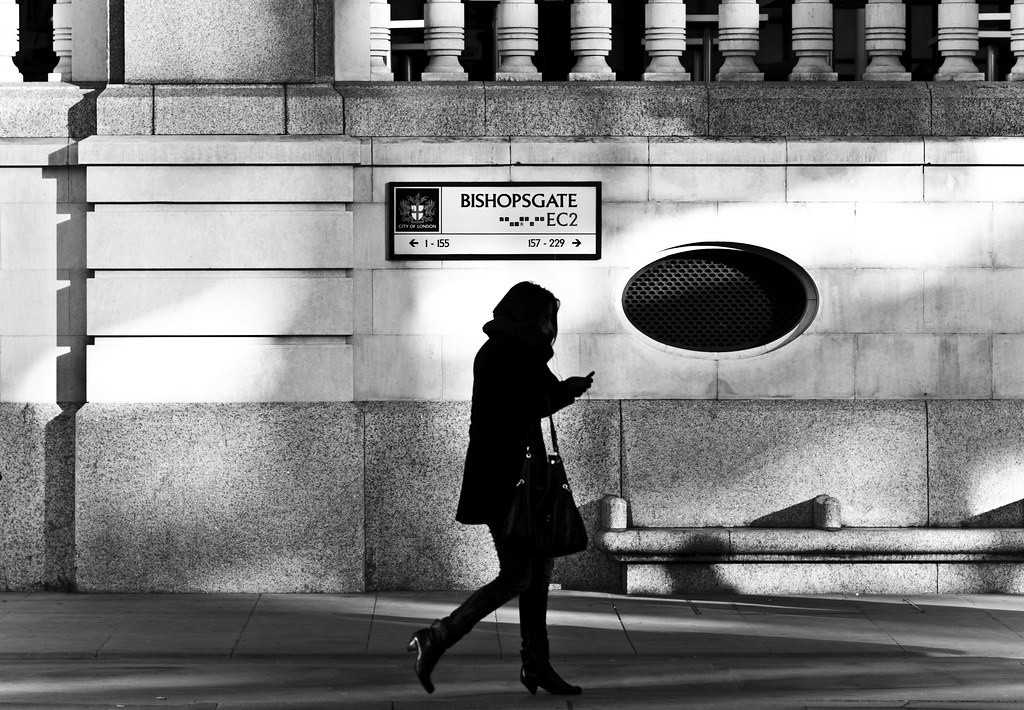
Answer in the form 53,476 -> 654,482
499,452 -> 588,558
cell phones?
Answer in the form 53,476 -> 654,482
586,371 -> 596,377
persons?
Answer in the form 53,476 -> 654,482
408,281 -> 593,697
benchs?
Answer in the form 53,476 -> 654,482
595,496 -> 1024,594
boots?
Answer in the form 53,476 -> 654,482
520,649 -> 583,695
407,619 -> 453,694
